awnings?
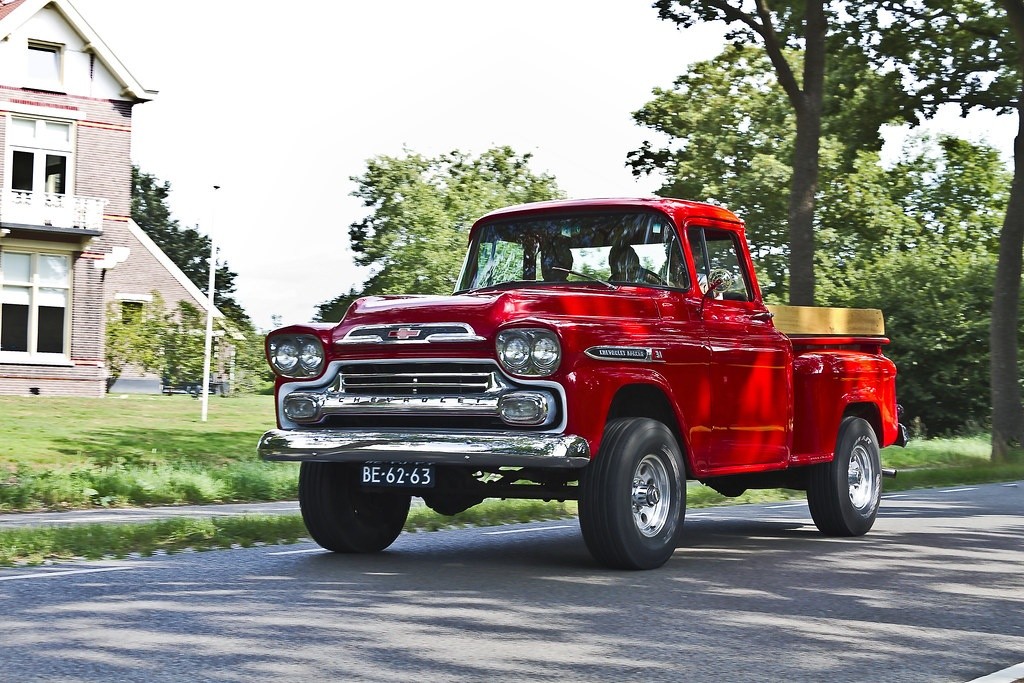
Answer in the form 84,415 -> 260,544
0,222 -> 102,236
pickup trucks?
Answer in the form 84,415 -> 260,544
254,197 -> 912,572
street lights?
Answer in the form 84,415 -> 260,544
201,183 -> 221,422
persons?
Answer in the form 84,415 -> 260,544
608,232 -> 724,300
541,247 -> 573,282
